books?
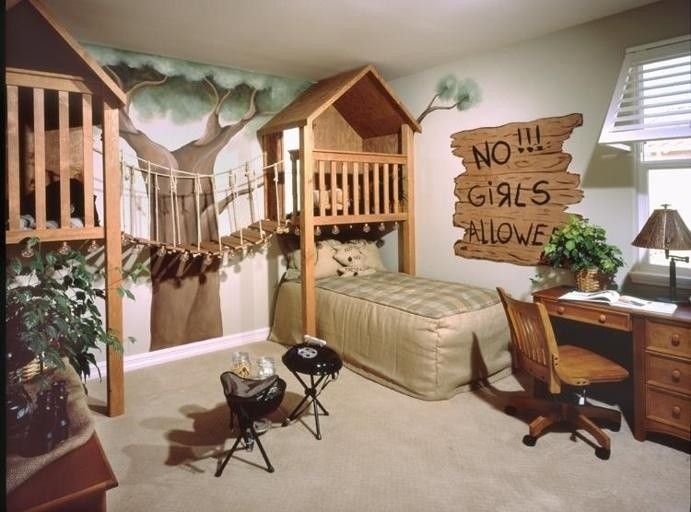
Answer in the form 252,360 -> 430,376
557,290 -> 678,316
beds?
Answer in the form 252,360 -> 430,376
268,274 -> 514,404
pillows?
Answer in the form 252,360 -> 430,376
282,235 -> 344,280
344,233 -> 388,273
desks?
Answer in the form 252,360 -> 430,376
529,283 -> 691,447
1,359 -> 120,512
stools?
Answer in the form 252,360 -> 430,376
210,369 -> 286,479
280,336 -> 345,443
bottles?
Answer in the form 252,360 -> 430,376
51,380 -> 72,442
256,356 -> 277,378
232,351 -> 253,379
31,390 -> 58,452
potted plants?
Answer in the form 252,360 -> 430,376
524,215 -> 630,295
4,234 -> 151,459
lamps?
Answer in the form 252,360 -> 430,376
631,201 -> 691,306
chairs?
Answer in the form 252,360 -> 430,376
495,282 -> 626,459
